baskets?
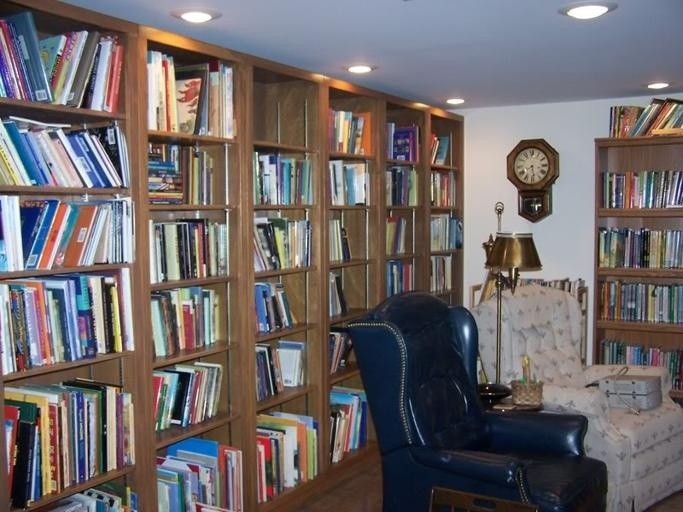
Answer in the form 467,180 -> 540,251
511,380 -> 544,406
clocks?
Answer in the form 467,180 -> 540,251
505,138 -> 558,222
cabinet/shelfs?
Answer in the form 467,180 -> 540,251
469,285 -> 592,368
2,1 -> 250,512
252,54 -> 464,512
592,134 -> 683,399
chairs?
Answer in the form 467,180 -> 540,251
471,278 -> 683,512
346,289 -> 608,512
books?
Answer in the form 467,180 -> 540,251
594,97 -> 683,389
520,277 -> 584,309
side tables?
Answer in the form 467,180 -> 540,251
492,398 -> 545,412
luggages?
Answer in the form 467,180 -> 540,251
599,375 -> 662,410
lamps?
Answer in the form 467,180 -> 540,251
646,81 -> 670,90
558,1 -> 616,20
485,231 -> 543,384
